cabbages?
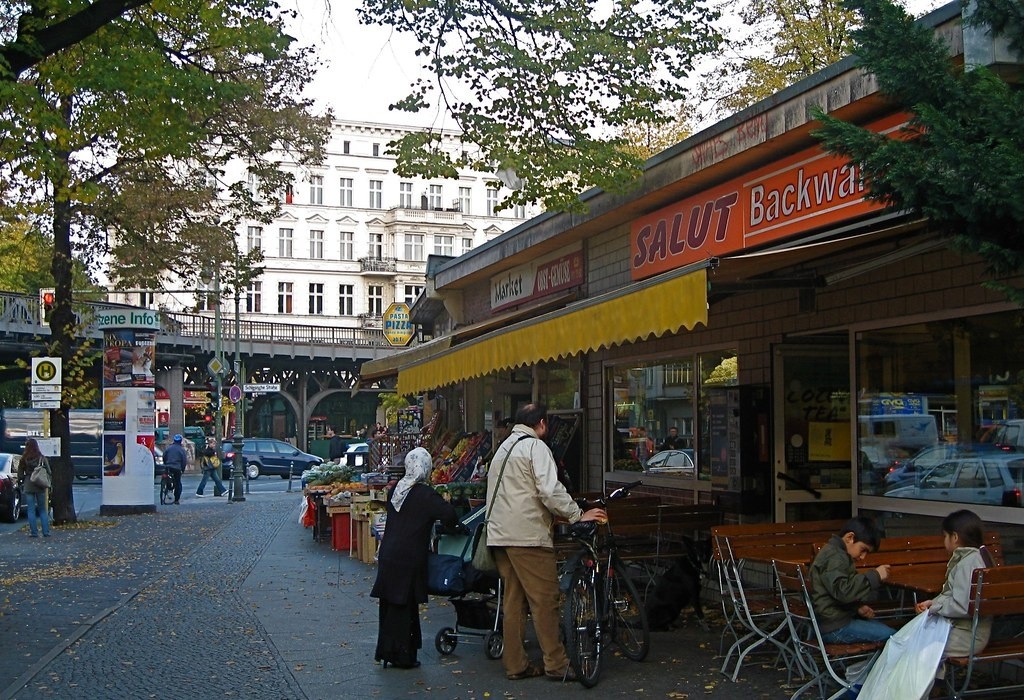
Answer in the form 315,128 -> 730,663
318,461 -> 342,472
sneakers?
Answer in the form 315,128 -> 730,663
508,660 -> 545,679
546,665 -> 578,680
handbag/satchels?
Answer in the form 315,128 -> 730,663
471,521 -> 498,575
30,457 -> 51,488
204,455 -> 220,469
423,550 -> 467,596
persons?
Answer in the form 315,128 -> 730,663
17,439 -> 53,537
660,427 -> 686,451
354,421 -> 388,447
327,425 -> 346,465
370,447 -> 471,670
162,433 -> 187,505
809,517 -> 898,645
195,437 -> 229,497
485,402 -> 608,681
916,509 -> 993,691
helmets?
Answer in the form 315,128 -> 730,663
174,434 -> 183,441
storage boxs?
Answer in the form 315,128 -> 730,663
431,430 -> 491,482
371,514 -> 387,526
322,498 -> 352,507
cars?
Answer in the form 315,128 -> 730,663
859,419 -> 1024,508
643,449 -> 697,475
221,436 -> 325,481
0,453 -> 52,523
338,441 -> 398,467
617,427 -> 653,464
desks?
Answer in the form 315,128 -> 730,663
747,554 -> 814,568
880,563 -> 947,615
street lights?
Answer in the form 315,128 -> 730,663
198,224 -> 247,501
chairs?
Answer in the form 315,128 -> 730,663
942,565 -> 1024,700
636,501 -> 724,606
768,557 -> 885,700
713,533 -> 809,684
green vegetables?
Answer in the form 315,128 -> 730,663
310,466 -> 357,485
435,482 -> 486,500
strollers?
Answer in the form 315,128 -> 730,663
431,521 -> 504,661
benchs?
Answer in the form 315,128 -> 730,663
709,518 -> 851,668
811,531 -> 1005,628
553,489 -> 683,561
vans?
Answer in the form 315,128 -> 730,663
856,414 -> 938,455
1,408 -> 166,477
154,426 -> 206,457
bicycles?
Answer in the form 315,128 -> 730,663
160,468 -> 185,505
556,479 -> 650,690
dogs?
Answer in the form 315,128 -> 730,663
617,534 -> 715,631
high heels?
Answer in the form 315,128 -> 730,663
382,659 -> 420,669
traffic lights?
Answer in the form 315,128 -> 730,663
205,380 -> 220,411
204,412 -> 212,435
40,287 -> 56,328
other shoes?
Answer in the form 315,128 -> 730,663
196,494 -> 205,497
221,490 -> 228,496
175,501 -> 179,504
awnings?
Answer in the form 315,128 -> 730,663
398,214 -> 962,398
358,292 -> 577,380
